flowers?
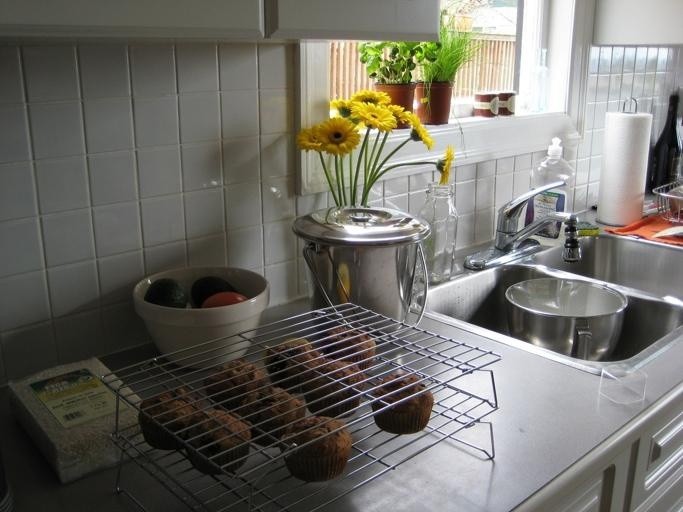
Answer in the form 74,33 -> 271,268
296,89 -> 454,206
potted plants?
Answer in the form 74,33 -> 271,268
356,0 -> 487,127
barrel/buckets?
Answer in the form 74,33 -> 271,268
301,234 -> 431,341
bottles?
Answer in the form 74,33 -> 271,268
416,178 -> 461,285
646,91 -> 682,197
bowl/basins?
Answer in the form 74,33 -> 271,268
502,276 -> 631,362
130,262 -> 271,372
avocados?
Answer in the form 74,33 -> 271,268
191,277 -> 238,307
145,278 -> 187,308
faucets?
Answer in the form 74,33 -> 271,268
493,180 -> 580,253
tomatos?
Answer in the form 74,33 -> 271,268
202,292 -> 248,308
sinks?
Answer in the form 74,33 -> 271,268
410,262 -> 683,379
518,232 -> 683,307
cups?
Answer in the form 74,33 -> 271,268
470,90 -> 519,118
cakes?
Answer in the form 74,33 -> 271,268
185,409 -> 250,476
371,374 -> 433,435
321,329 -> 376,370
241,384 -> 306,445
304,358 -> 366,420
265,337 -> 324,395
139,388 -> 204,451
278,416 -> 353,481
204,360 -> 271,416
